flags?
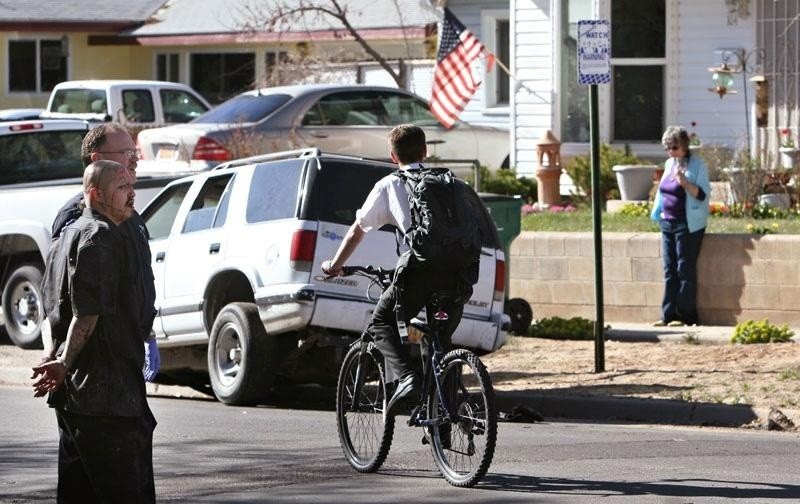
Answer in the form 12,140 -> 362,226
428,8 -> 495,130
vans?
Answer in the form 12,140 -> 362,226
40,80 -> 212,125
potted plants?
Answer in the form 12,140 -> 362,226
759,182 -> 792,213
614,155 -> 657,203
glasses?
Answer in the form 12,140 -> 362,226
97,148 -> 142,160
664,145 -> 679,150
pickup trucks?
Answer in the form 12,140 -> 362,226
0,119 -> 200,349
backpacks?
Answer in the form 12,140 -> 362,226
393,168 -> 481,284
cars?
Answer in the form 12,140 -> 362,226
136,83 -> 510,180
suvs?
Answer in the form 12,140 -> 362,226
138,148 -> 506,405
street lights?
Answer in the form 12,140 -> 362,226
708,45 -> 767,168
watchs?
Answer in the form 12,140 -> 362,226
329,262 -> 339,271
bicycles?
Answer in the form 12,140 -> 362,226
323,265 -> 498,487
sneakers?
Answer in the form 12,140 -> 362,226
667,319 -> 684,327
653,319 -> 666,326
385,372 -> 419,418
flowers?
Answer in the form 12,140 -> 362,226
780,129 -> 795,151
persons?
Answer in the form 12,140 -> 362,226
32,160 -> 155,504
51,121 -> 156,503
321,124 -> 480,449
649,125 -> 711,327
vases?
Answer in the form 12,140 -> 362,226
781,143 -> 794,173
723,165 -> 765,207
